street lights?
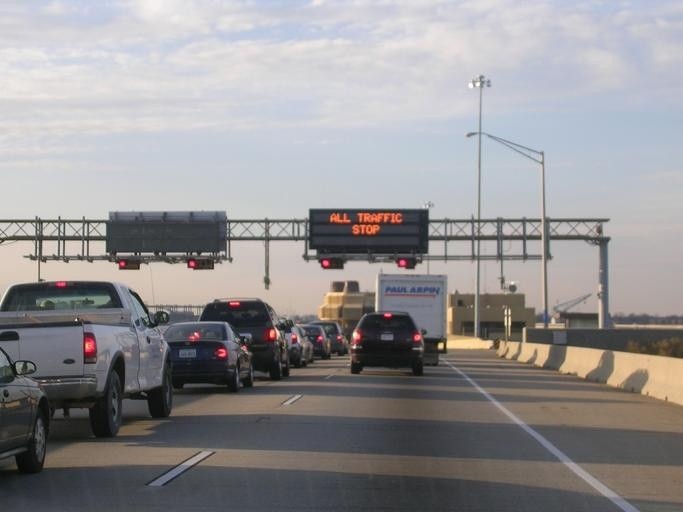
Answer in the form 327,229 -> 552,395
464,130 -> 548,326
423,200 -> 435,274
468,72 -> 491,338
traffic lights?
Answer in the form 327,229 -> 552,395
320,258 -> 343,269
186,258 -> 214,269
396,257 -> 416,269
117,260 -> 139,269
